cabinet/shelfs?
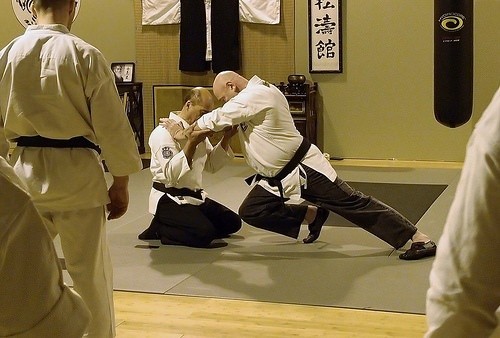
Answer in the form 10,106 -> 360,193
117,79 -> 146,154
284,84 -> 321,150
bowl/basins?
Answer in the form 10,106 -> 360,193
288,75 -> 306,84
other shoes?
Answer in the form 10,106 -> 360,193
302,206 -> 330,244
138,217 -> 159,239
399,239 -> 437,261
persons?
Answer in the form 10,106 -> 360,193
158,71 -> 437,259
0,158 -> 93,338
425,87 -> 500,338
112,64 -> 131,82
138,87 -> 242,248
0,0 -> 143,338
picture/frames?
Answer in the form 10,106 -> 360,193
152,84 -> 245,158
